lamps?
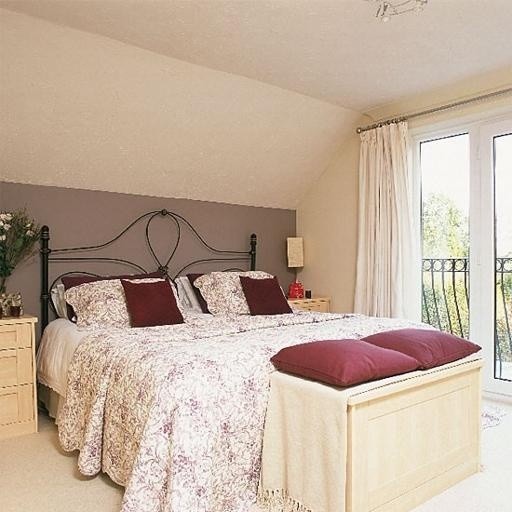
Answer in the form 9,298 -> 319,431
285,236 -> 304,298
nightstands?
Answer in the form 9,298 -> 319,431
0,313 -> 38,442
285,295 -> 330,314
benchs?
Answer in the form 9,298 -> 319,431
271,353 -> 481,512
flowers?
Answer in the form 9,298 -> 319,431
0,203 -> 43,296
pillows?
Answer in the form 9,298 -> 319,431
270,329 -> 481,389
58,269 -> 291,332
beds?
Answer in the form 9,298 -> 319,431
36,207 -> 486,511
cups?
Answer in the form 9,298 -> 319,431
10,305 -> 22,318
304,289 -> 312,298
